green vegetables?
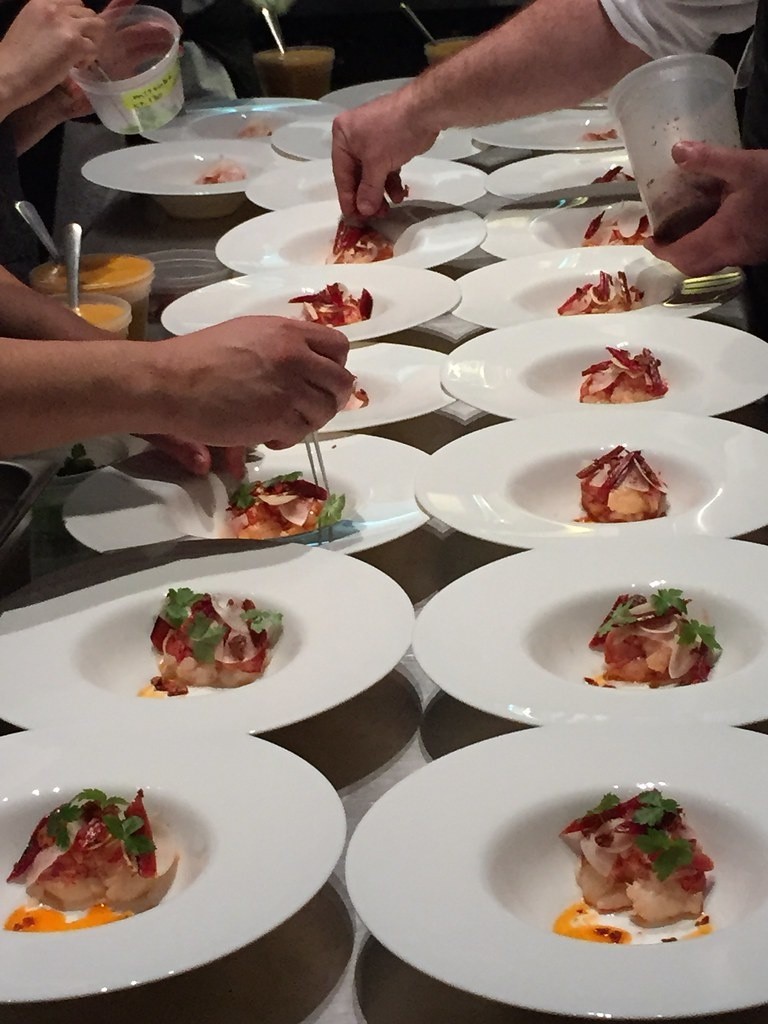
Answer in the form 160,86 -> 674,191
228,471 -> 347,526
47,788 -> 162,859
162,587 -> 283,660
582,789 -> 697,883
594,589 -> 723,649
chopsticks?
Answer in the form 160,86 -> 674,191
302,431 -> 333,499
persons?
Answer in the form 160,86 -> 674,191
0,0 -> 185,289
331,1 -> 768,286
0,268 -> 357,482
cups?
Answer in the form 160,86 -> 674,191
30,252 -> 156,345
607,53 -> 746,245
424,35 -> 476,67
136,247 -> 231,342
251,45 -> 335,100
68,4 -> 186,136
46,292 -> 132,339
8,434 -> 130,584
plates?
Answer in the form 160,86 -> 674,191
0,78 -> 768,1024
